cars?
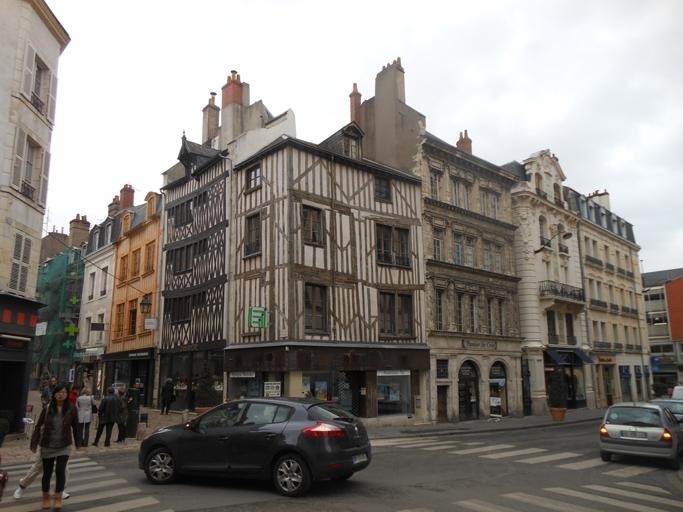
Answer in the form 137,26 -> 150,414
599,385 -> 682,470
108,379 -> 128,391
375,381 -> 406,402
137,397 -> 373,498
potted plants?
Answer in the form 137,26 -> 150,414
549,372 -> 567,422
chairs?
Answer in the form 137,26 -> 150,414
16,404 -> 36,440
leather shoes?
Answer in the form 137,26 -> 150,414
84,437 -> 125,447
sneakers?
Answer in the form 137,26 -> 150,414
50,489 -> 71,499
13,484 -> 25,500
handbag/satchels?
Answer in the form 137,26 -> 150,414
91,395 -> 98,413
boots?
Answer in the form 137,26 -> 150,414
41,490 -> 52,509
53,491 -> 63,509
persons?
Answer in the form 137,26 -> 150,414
160,377 -> 174,415
666,386 -> 672,399
91,387 -> 117,446
39,376 -> 57,407
303,391 -> 314,402
10,449 -> 69,499
74,386 -> 96,447
112,387 -> 128,443
67,384 -> 79,403
29,382 -> 82,509
